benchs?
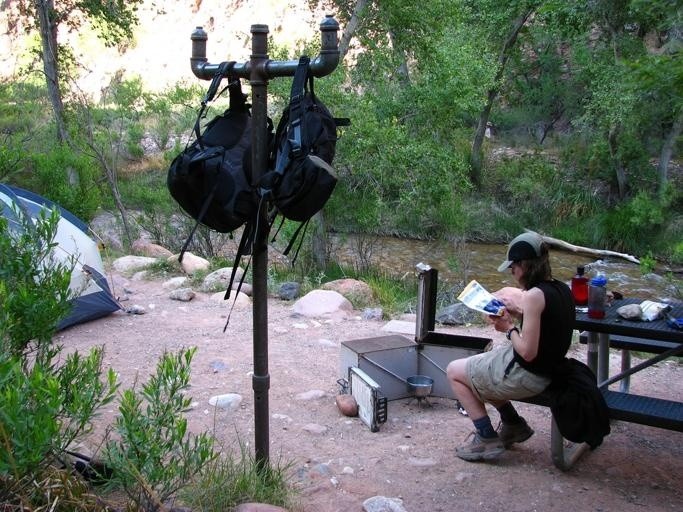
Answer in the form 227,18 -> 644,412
579,330 -> 683,395
506,384 -> 683,470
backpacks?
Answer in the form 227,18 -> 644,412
166,60 -> 274,233
256,56 -> 339,223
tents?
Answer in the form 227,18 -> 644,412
1,182 -> 126,336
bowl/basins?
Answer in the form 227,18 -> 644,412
405,375 -> 434,397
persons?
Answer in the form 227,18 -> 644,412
446,232 -> 576,463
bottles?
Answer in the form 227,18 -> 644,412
588,276 -> 606,319
571,263 -> 590,313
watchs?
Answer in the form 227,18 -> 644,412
505,326 -> 519,340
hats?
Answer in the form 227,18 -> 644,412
497,232 -> 549,274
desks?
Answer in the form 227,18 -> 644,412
574,293 -> 683,396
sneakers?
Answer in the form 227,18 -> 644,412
497,416 -> 535,443
453,433 -> 506,461
